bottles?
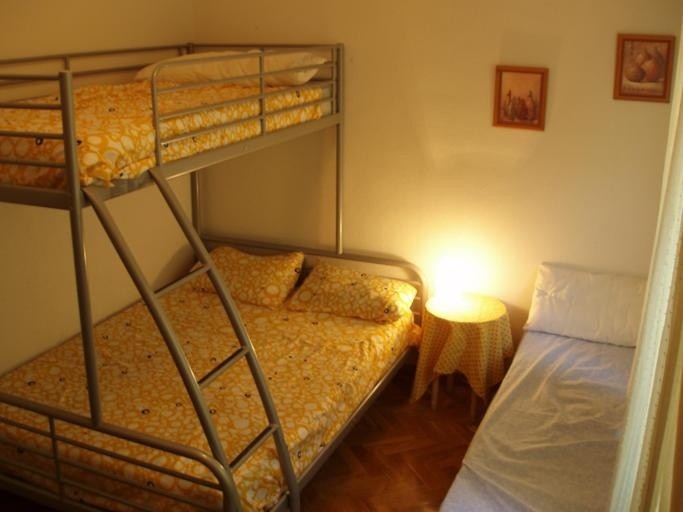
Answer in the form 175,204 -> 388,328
502,87 -> 536,123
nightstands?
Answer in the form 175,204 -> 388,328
410,294 -> 515,422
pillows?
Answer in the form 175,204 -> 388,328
522,264 -> 646,347
189,247 -> 305,309
287,263 -> 417,323
133,48 -> 327,86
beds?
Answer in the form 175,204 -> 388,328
1,43 -> 428,512
439,330 -> 635,512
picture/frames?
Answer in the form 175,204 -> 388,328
613,33 -> 674,103
492,65 -> 549,131
